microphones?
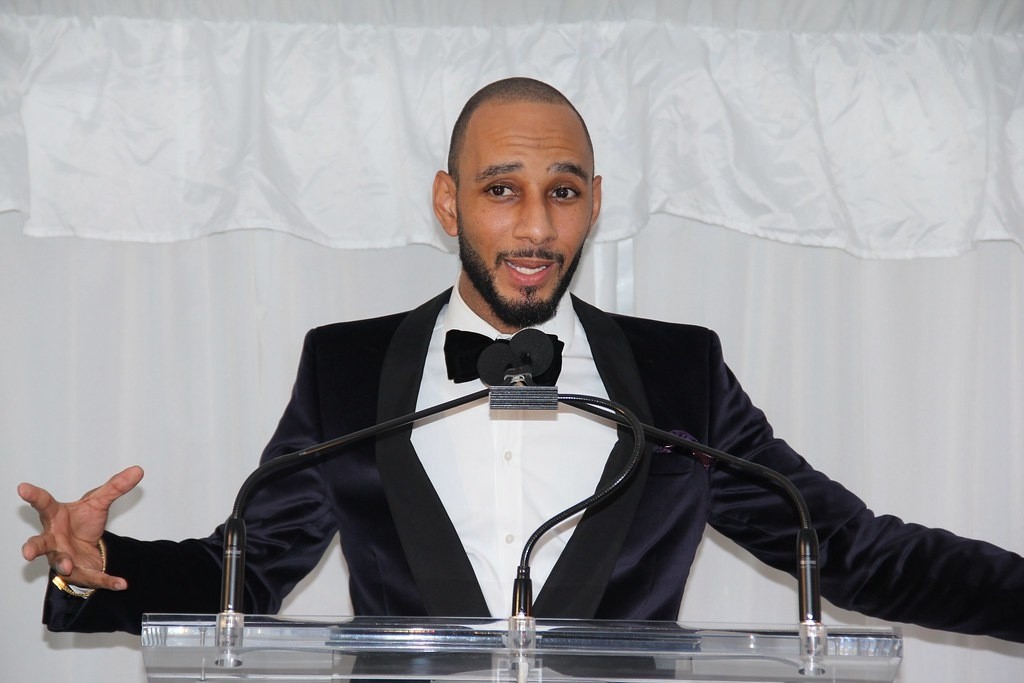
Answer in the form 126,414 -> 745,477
220,329 -> 823,629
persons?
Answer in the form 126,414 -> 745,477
15,73 -> 1024,645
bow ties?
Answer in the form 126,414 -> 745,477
444,328 -> 564,388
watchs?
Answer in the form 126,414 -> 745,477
48,536 -> 108,597
